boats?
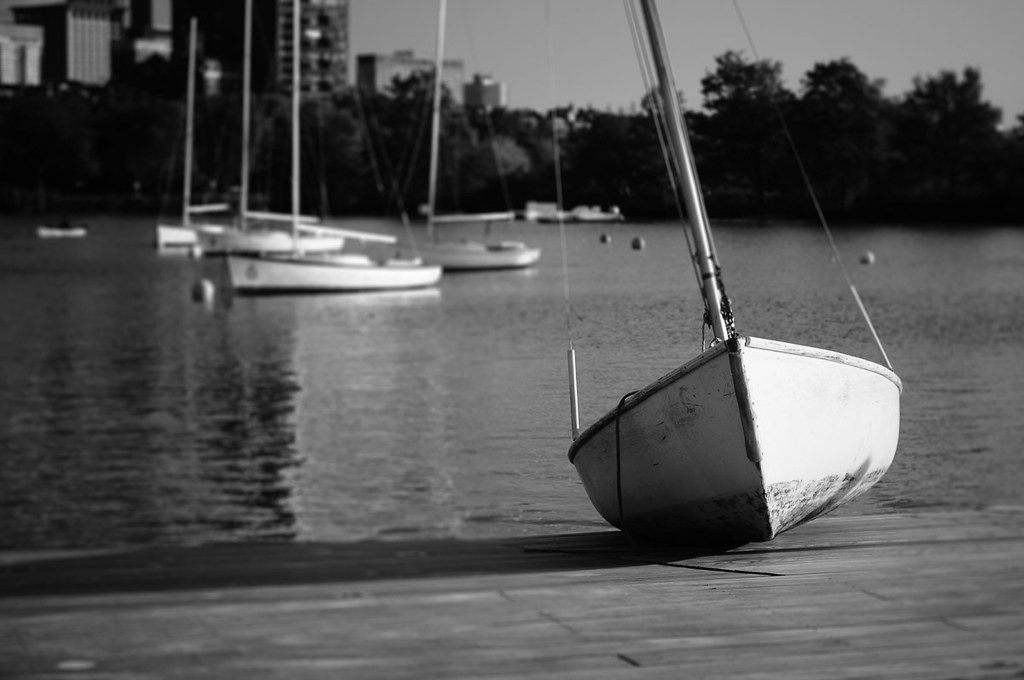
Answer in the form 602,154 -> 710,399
33,225 -> 88,240
429,199 -> 626,231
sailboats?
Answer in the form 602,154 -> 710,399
152,1 -> 351,260
396,0 -> 541,273
536,0 -> 904,554
217,0 -> 446,295
